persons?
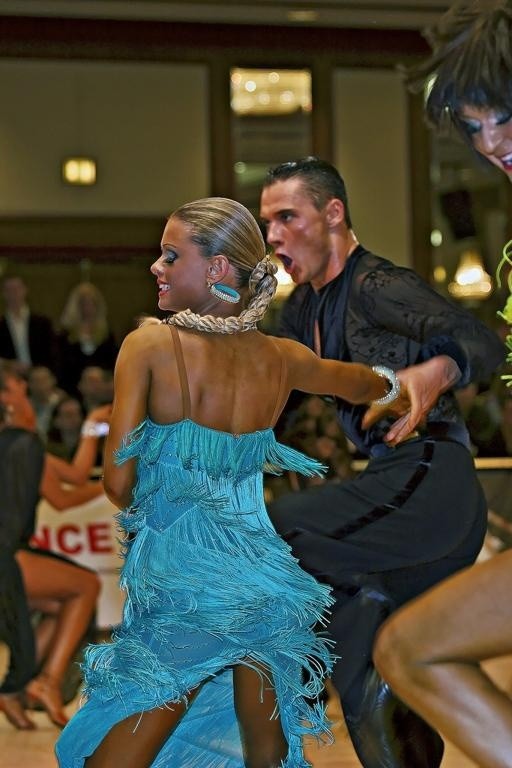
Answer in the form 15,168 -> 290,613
51,283 -> 122,400
374,0 -> 512,768
259,158 -> 506,768
54,197 -> 428,768
1,266 -> 55,379
1,329 -> 512,492
0,371 -> 112,732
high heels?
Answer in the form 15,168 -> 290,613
24,673 -> 68,730
